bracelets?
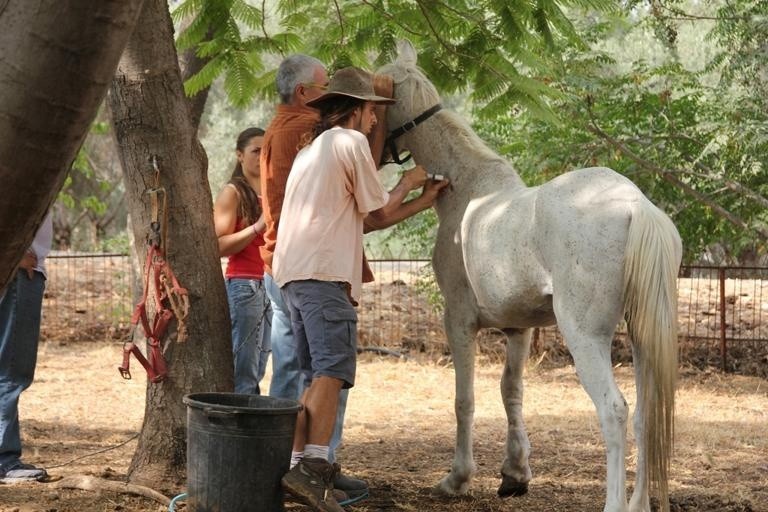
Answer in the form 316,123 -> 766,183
252,224 -> 260,238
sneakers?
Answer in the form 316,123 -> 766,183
329,462 -> 369,494
279,457 -> 345,512
0,463 -> 47,484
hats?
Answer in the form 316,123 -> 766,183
306,67 -> 397,106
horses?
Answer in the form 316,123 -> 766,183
367,37 -> 683,512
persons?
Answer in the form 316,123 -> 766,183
0,206 -> 53,484
259,53 -> 395,505
214,127 -> 274,394
270,68 -> 452,510
